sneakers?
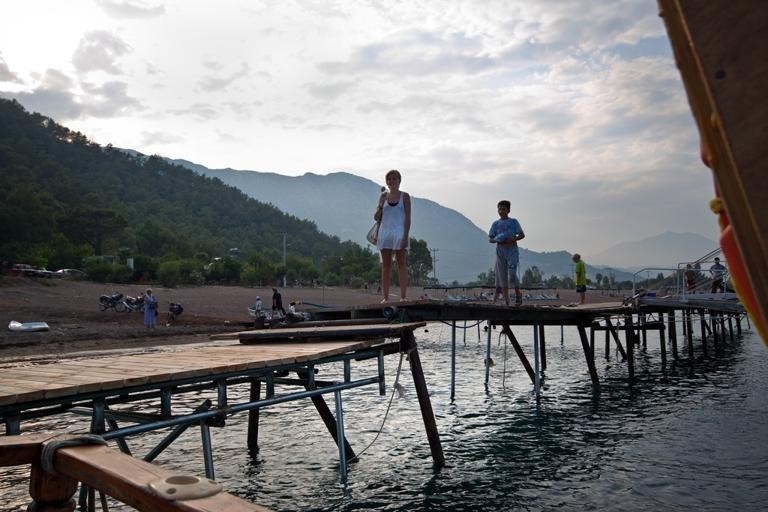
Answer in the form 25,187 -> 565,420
380,298 -> 387,303
400,297 -> 406,302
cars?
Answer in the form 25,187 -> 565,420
11,262 -> 84,278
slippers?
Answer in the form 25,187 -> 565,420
515,293 -> 522,306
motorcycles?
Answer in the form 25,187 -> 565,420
98,292 -> 127,313
123,292 -> 145,313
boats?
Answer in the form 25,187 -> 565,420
249,305 -> 312,320
424,287 -> 560,302
593,316 -> 662,330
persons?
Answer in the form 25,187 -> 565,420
144,288 -> 158,332
489,262 -> 501,305
375,279 -> 382,296
288,302 -> 299,314
256,296 -> 262,319
488,201 -> 525,306
571,253 -> 586,305
709,258 -> 729,293
373,169 -> 411,303
684,264 -> 697,294
271,287 -> 286,315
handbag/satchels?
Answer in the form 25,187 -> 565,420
366,222 -> 379,245
